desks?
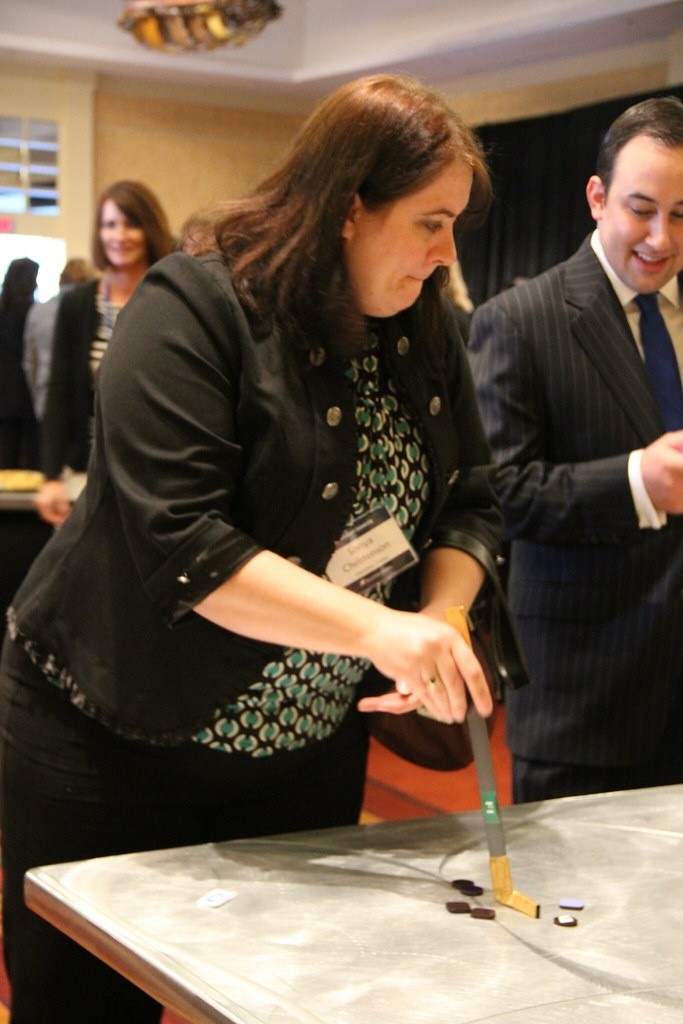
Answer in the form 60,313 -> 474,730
24,784 -> 683,1024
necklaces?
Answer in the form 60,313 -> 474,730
105,270 -> 114,333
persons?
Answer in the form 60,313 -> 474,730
465,98 -> 683,807
0,72 -> 507,1023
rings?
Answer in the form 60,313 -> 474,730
425,676 -> 440,689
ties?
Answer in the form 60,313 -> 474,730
634,293 -> 683,433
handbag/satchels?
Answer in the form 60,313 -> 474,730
367,596 -> 496,771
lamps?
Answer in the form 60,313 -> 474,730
116,0 -> 285,57
